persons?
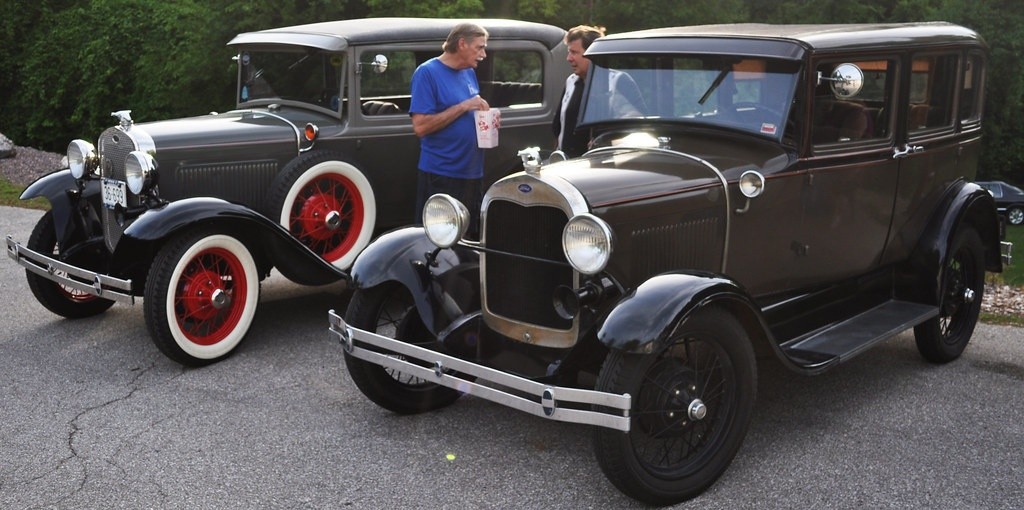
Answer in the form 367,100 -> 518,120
409,24 -> 500,223
553,25 -> 649,159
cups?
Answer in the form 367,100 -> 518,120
474,107 -> 500,149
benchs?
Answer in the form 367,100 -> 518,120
479,75 -> 541,108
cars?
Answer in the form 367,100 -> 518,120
329,22 -> 1010,508
975,182 -> 1024,226
5,15 -> 567,367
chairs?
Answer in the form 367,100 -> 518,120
813,100 -> 872,144
259,98 -> 360,107
